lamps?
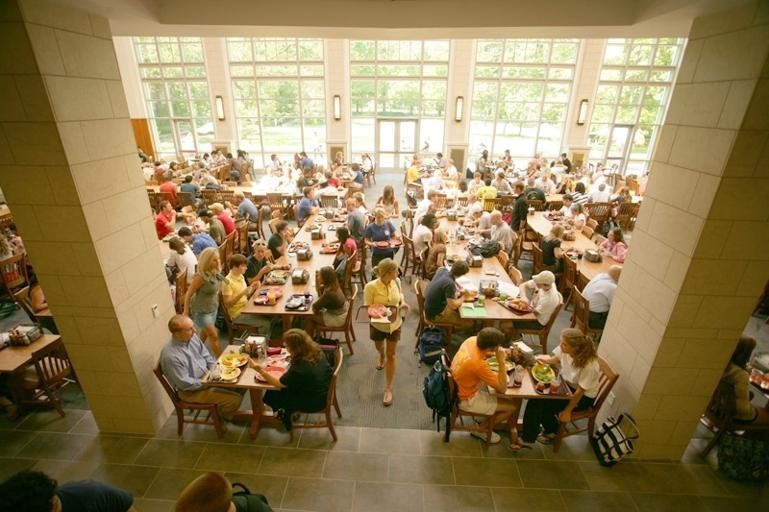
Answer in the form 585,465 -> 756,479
577,98 -> 589,126
215,94 -> 225,120
333,94 -> 341,120
455,95 -> 464,122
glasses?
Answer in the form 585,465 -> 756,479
389,270 -> 398,273
257,243 -> 266,247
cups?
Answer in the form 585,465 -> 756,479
268,293 -> 275,304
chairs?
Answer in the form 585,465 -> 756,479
0,150 -> 768,460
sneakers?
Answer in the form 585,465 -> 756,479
471,429 -> 501,443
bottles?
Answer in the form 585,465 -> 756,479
6,328 -> 31,346
304,291 -> 312,312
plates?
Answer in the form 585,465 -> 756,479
266,286 -> 282,298
208,344 -> 292,383
505,300 -> 531,312
254,295 -> 268,303
285,297 -> 302,309
485,351 -> 563,398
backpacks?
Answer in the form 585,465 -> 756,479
424,364 -> 457,433
415,328 -> 447,364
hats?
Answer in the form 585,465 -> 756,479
230,171 -> 239,177
199,210 -> 211,218
533,270 -> 556,284
428,190 -> 439,197
208,203 -> 224,211
469,205 -> 481,214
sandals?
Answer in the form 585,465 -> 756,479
376,360 -> 384,371
537,435 -> 556,443
510,443 -> 532,450
383,388 -> 394,405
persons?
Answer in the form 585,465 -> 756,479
406,148 -> 650,329
362,258 -> 407,406
159,314 -> 242,430
715,336 -> 769,427
1,220 -> 64,422
138,145 -> 406,358
0,468 -> 137,512
517,329 -> 601,451
173,471 -> 273,511
246,329 -> 333,431
448,326 -> 516,444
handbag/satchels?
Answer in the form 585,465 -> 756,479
334,253 -> 347,275
473,241 -> 501,256
312,334 -> 338,367
590,414 -> 640,466
